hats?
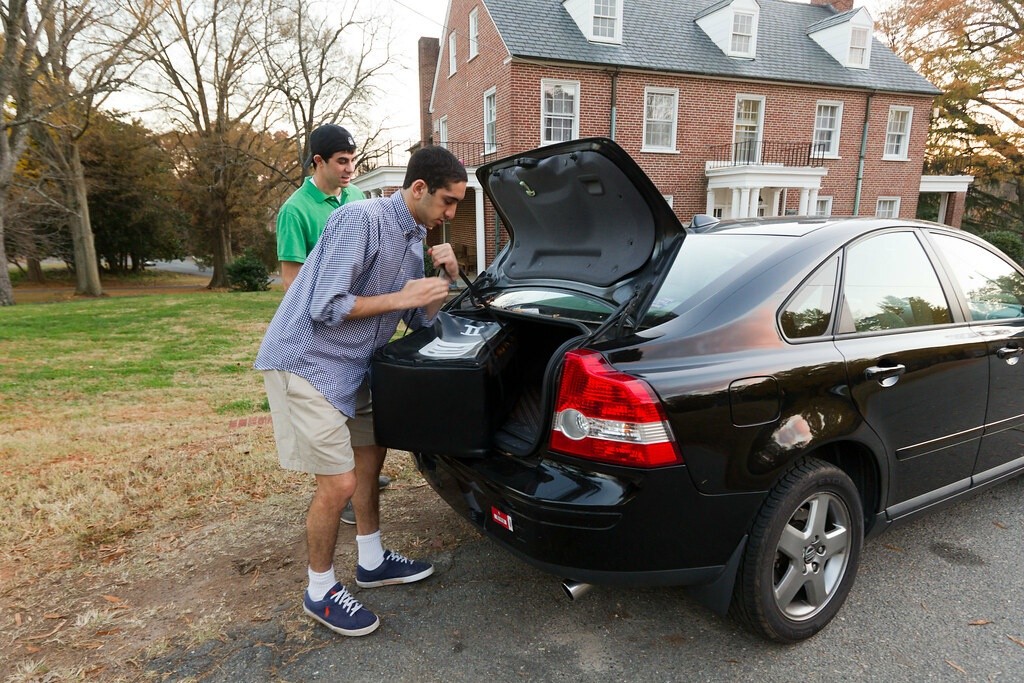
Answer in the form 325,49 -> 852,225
303,124 -> 357,169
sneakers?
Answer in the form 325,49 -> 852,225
355,550 -> 435,589
339,502 -> 356,525
379,475 -> 390,490
302,581 -> 379,637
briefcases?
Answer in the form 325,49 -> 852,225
370,260 -> 516,455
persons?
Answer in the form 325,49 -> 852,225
251,144 -> 469,638
274,124 -> 389,525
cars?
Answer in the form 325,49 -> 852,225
405,134 -> 1024,646
